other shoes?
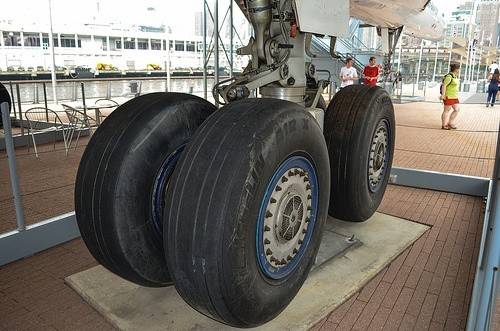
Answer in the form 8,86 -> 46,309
442,125 -> 450,130
491,103 -> 494,107
486,102 -> 489,108
447,123 -> 456,129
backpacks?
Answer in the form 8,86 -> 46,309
440,72 -> 455,94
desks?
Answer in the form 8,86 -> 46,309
73,105 -> 118,125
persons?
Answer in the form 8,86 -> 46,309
339,58 -> 358,89
363,57 -> 379,86
439,63 -> 460,130
486,61 -> 500,107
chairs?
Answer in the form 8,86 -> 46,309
25,107 -> 67,159
61,103 -> 101,151
94,99 -> 119,127
